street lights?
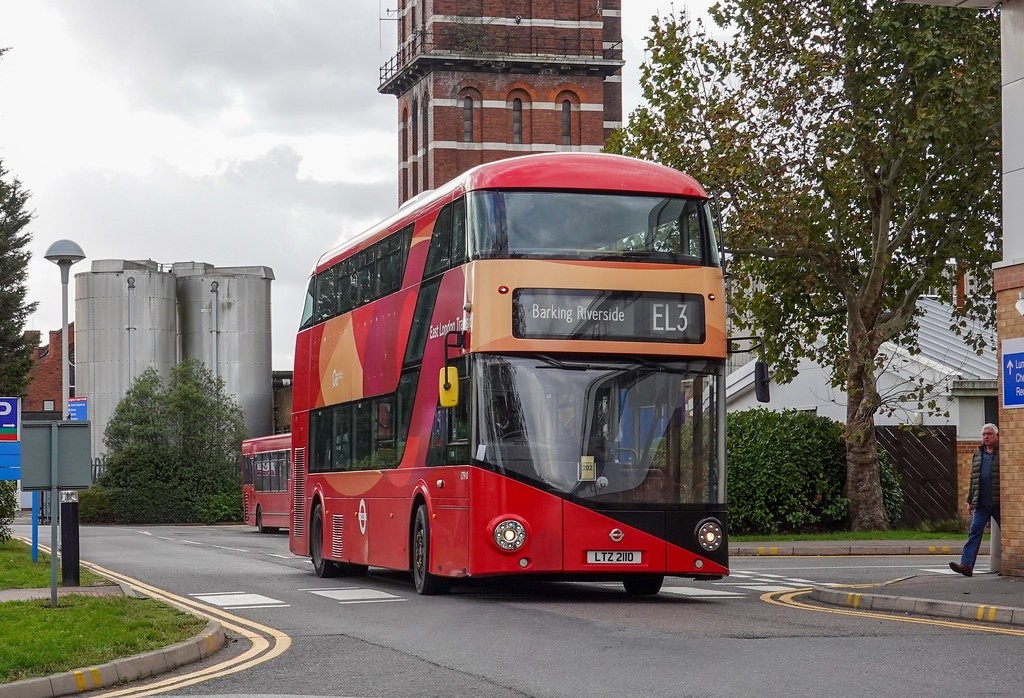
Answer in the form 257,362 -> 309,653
41,238 -> 86,586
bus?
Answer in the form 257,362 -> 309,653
289,149 -> 770,596
239,432 -> 295,534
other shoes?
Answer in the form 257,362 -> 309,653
949,561 -> 973,577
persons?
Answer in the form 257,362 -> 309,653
949,423 -> 1002,577
